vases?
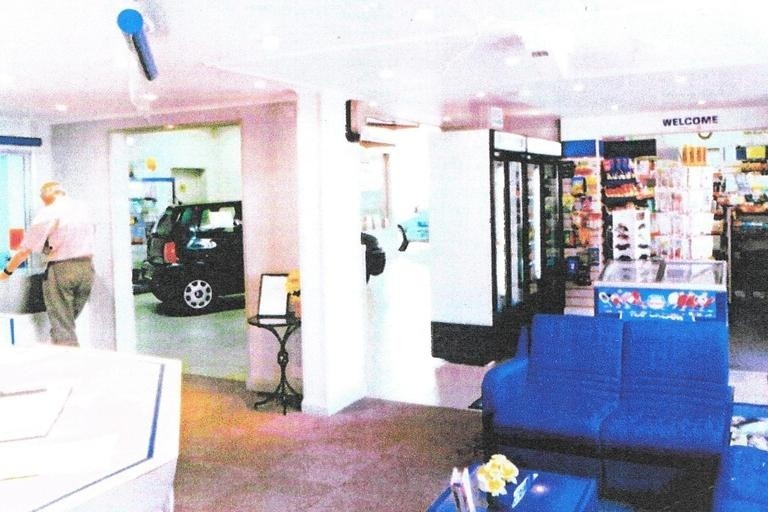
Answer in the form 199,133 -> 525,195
285,292 -> 302,325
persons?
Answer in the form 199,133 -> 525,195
0,180 -> 95,348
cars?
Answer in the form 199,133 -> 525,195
141,199 -> 244,314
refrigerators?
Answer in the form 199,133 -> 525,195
594,259 -> 728,324
430,127 -> 566,367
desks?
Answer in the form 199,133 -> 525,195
248,313 -> 301,419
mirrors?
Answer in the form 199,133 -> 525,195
0,147 -> 38,276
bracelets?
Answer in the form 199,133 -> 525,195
3,268 -> 12,276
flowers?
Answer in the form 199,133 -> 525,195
284,268 -> 301,304
474,448 -> 519,494
